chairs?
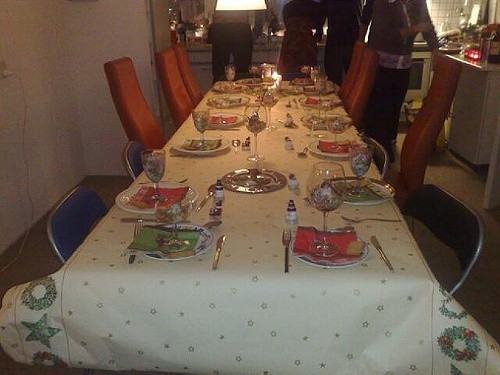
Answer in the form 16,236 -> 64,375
47,41 -> 484,294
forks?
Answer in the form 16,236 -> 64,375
282,228 -> 292,274
341,216 -> 402,223
129,223 -> 143,264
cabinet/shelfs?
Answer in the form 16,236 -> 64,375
448,64 -> 500,164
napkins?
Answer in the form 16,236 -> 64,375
214,97 -> 242,105
180,138 -> 221,151
292,77 -> 314,86
335,181 -> 391,203
208,115 -> 237,125
128,225 -> 203,256
317,141 -> 357,154
305,97 -> 330,108
293,225 -> 363,264
127,185 -> 190,207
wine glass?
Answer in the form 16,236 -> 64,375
139,148 -> 167,203
191,108 -> 209,148
244,62 -> 281,162
300,63 -> 373,195
224,64 -> 235,85
306,161 -> 346,257
155,196 -> 189,248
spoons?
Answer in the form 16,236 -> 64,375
202,221 -> 222,228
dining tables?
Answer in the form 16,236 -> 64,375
0,80 -> 500,375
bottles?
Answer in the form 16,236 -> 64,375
285,137 -> 299,231
214,176 -> 224,203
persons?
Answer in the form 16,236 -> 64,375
212,11 -> 263,85
277,0 -> 319,81
360,0 -> 439,162
314,0 -> 364,86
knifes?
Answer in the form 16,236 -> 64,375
371,236 -> 394,272
120,218 -> 192,223
213,234 -> 225,270
195,184 -> 215,212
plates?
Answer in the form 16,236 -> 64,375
288,229 -> 369,269
221,169 -> 287,194
115,112 -> 246,261
290,77 -> 396,205
206,76 -> 274,109
